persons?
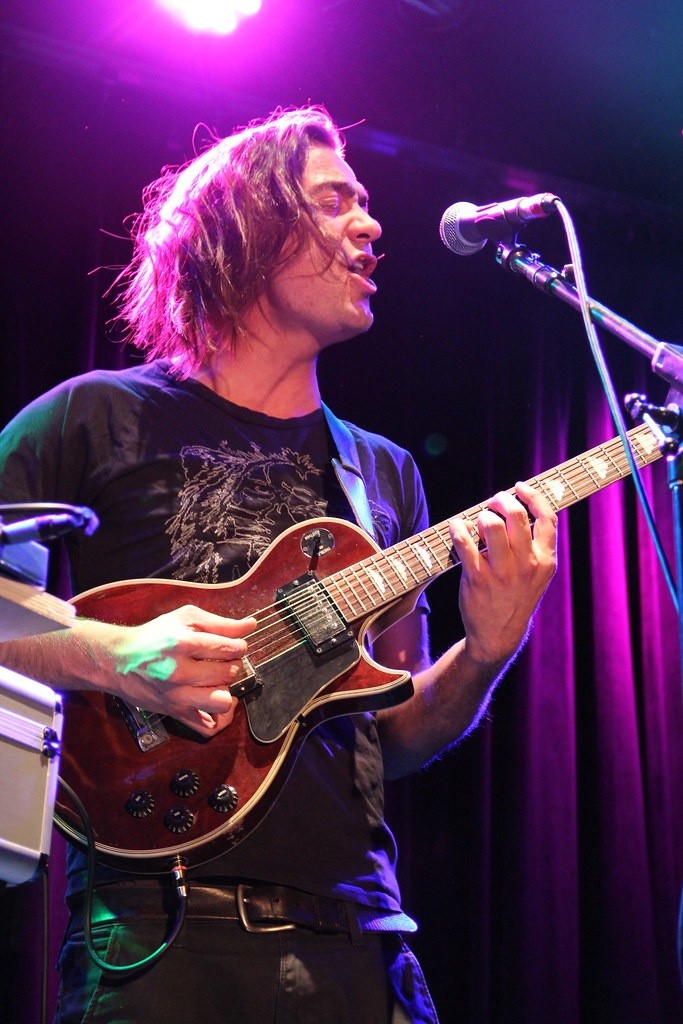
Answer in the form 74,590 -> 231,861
1,101 -> 561,1024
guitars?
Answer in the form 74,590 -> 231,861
48,383 -> 683,873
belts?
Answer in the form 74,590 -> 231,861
63,878 -> 418,934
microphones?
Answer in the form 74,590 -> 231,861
440,193 -> 557,256
